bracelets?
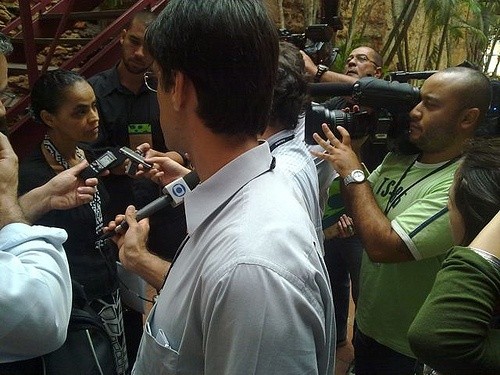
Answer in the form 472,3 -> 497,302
468,247 -> 500,271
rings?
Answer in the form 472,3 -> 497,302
348,227 -> 352,233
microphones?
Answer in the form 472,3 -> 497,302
306,82 -> 353,97
100,172 -> 201,240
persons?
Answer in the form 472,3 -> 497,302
0,34 -> 96,375
16,69 -> 129,375
103,0 -> 337,375
84,8 -> 186,316
257,41 -> 500,375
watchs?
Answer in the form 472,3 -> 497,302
313,63 -> 329,82
343,170 -> 367,186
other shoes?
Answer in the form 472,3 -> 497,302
338,335 -> 348,346
347,359 -> 357,375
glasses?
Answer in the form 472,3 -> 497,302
344,54 -> 379,69
143,55 -> 160,93
0,90 -> 16,111
